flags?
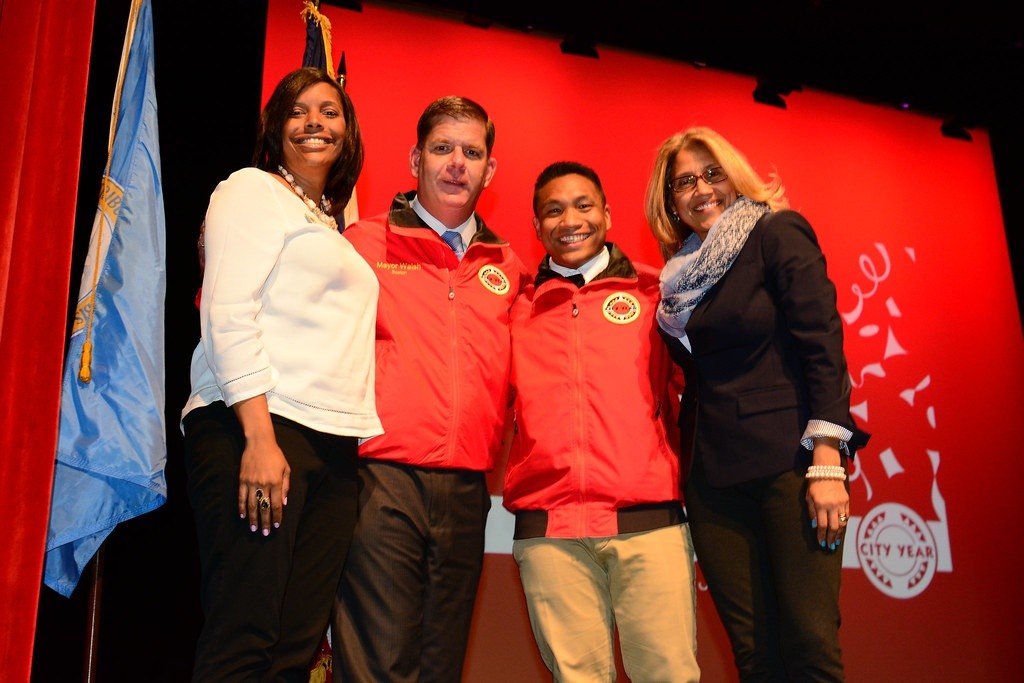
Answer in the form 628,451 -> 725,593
42,0 -> 166,599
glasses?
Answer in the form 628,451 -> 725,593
667,167 -> 728,192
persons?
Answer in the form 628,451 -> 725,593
194,97 -> 534,683
639,124 -> 860,683
180,66 -> 385,683
498,160 -> 702,683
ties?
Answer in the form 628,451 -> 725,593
440,231 -> 465,261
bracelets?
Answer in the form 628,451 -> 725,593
806,465 -> 848,482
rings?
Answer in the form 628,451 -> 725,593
258,496 -> 270,510
199,242 -> 204,247
839,513 -> 849,523
255,488 -> 264,502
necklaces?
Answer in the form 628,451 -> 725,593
276,163 -> 339,231
